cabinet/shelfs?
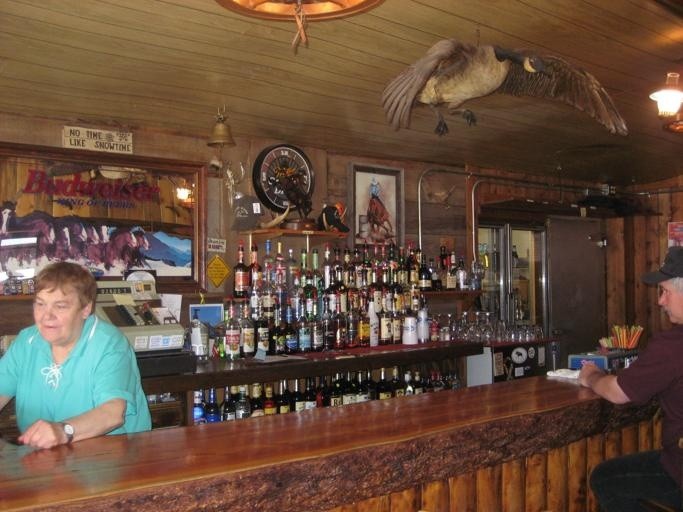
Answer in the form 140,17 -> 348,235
170,288 -> 560,427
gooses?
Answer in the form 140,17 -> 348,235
378,37 -> 628,138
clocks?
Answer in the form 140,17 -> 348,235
252,146 -> 315,210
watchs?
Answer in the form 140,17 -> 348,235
57,421 -> 76,447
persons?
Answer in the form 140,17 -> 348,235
1,263 -> 151,448
579,245 -> 683,512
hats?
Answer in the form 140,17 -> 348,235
639,245 -> 683,285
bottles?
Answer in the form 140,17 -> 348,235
191,369 -> 377,425
377,366 -> 461,400
217,234 -> 435,361
438,243 -> 499,290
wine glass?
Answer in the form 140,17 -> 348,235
435,310 -> 543,345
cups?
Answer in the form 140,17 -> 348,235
145,391 -> 176,405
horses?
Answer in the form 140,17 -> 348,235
367,194 -> 393,234
0,199 -> 151,276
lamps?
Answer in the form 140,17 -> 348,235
650,72 -> 683,132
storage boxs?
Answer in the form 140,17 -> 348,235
567,349 -> 640,373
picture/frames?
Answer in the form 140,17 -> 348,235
0,143 -> 205,297
348,162 -> 405,249
